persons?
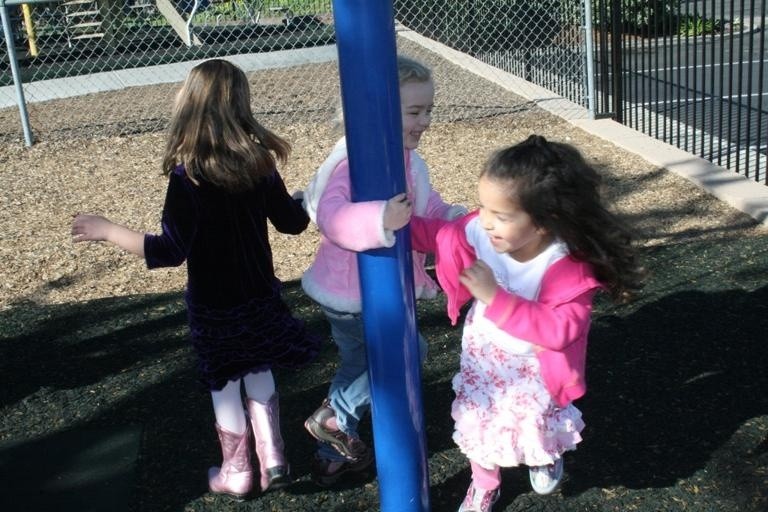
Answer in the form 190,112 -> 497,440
69,57 -> 310,500
409,133 -> 644,512
300,51 -> 470,474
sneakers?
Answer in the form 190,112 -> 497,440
304,397 -> 374,488
459,480 -> 502,512
528,452 -> 564,496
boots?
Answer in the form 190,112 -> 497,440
206,410 -> 252,503
243,392 -> 295,494
